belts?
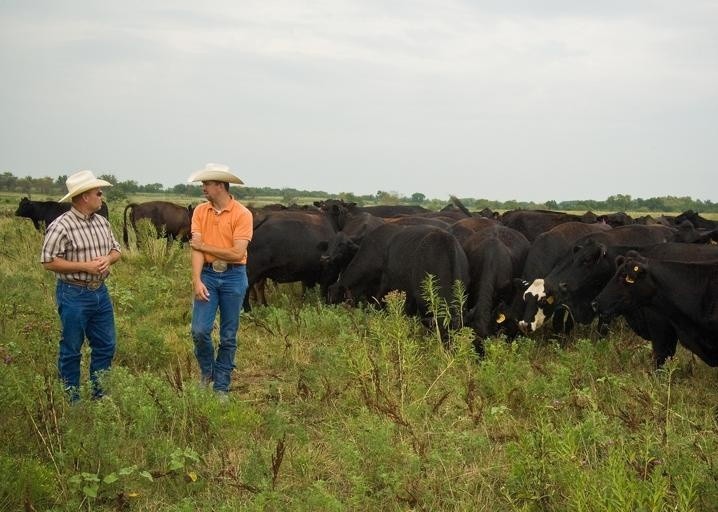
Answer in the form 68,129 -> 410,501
201,260 -> 243,272
60,277 -> 104,291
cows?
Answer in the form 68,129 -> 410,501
123,202 -> 195,253
243,195 -> 718,370
14,197 -> 108,236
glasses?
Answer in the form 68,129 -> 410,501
90,190 -> 104,199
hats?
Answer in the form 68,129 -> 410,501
191,162 -> 245,185
58,169 -> 114,205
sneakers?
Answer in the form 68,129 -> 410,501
198,376 -> 213,394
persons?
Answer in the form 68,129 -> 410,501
39,168 -> 123,407
186,162 -> 254,400
